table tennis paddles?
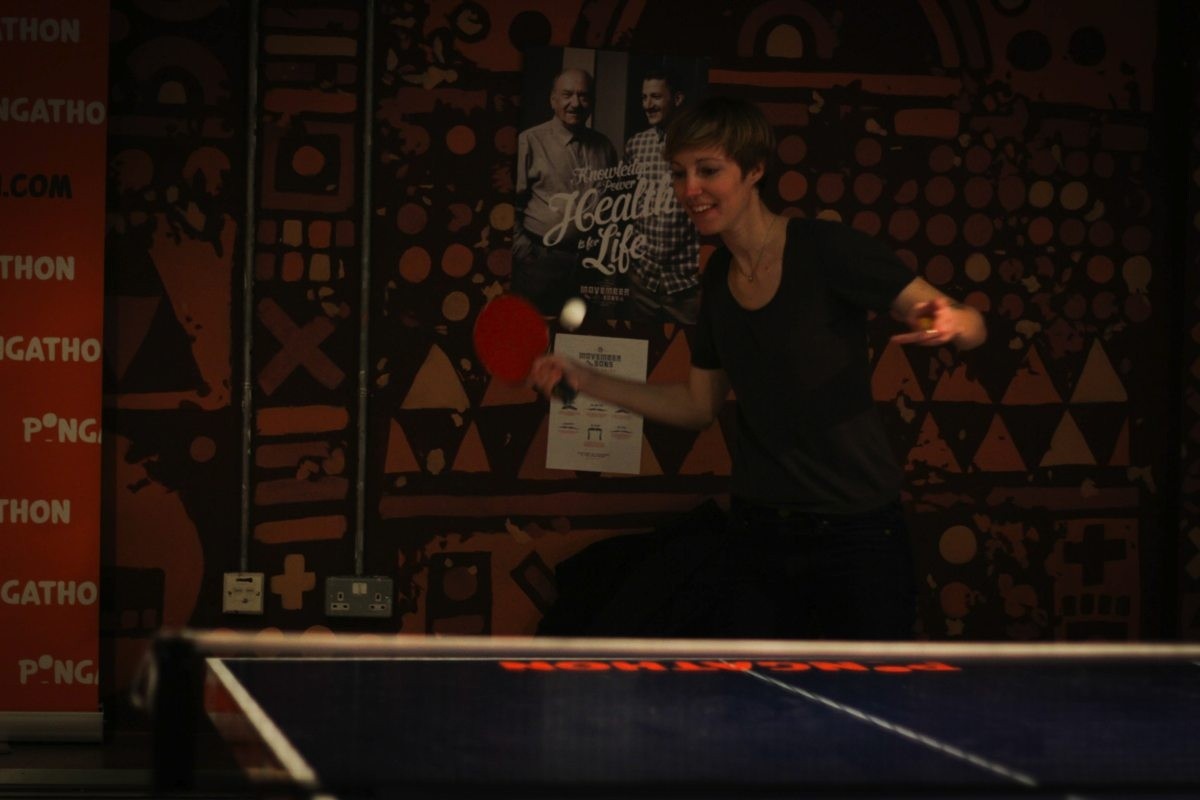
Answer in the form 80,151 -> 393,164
475,294 -> 578,404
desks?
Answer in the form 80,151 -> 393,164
146,629 -> 1199,787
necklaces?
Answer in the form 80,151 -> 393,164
737,214 -> 776,282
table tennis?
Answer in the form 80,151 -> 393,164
559,298 -> 586,329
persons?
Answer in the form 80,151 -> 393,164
529,94 -> 987,642
516,69 -> 620,316
620,62 -> 702,337
